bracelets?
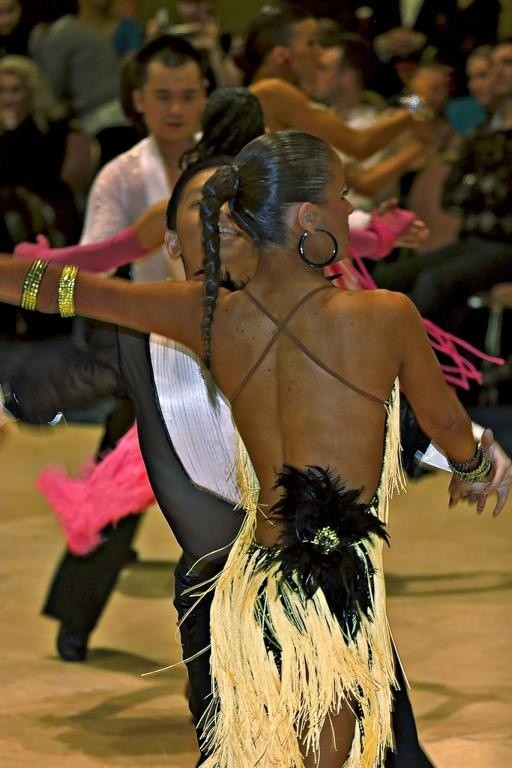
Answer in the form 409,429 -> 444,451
57,255 -> 78,321
446,442 -> 488,481
19,249 -> 45,310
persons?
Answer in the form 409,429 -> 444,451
2,0 -> 511,478
36,37 -> 214,661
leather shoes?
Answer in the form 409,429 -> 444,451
59,628 -> 91,660
